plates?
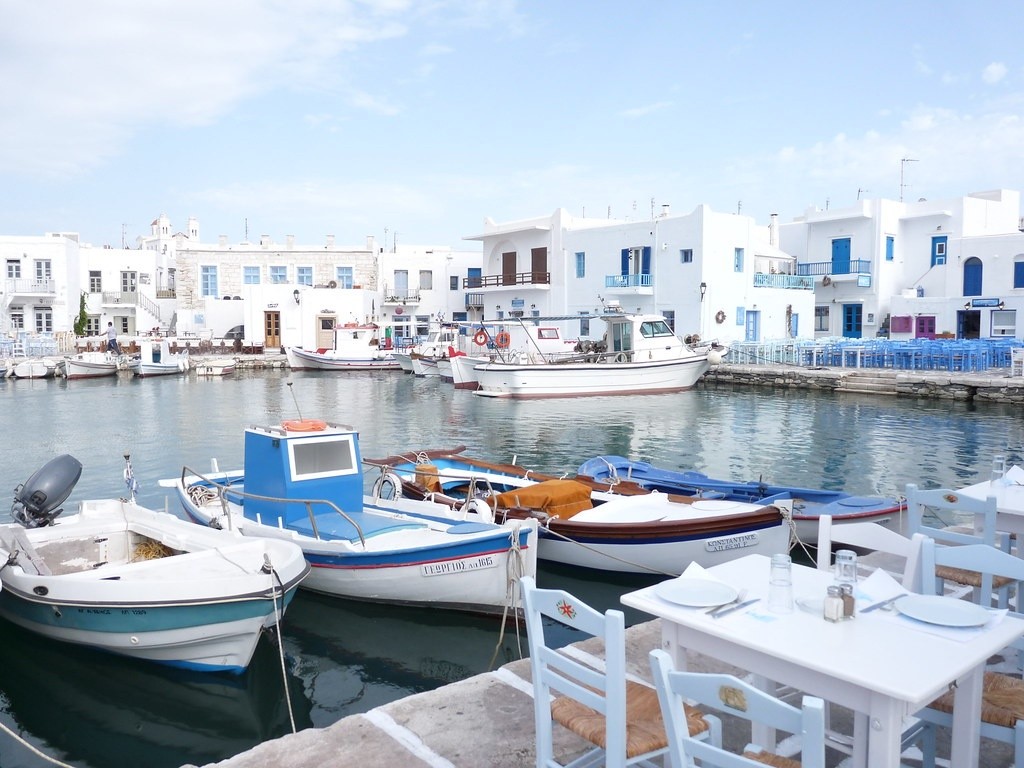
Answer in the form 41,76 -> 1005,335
895,595 -> 990,627
655,579 -> 738,606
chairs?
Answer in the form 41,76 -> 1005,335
520,483 -> 1024,768
614,275 -> 627,288
0,332 -> 74,357
728,336 -> 1024,376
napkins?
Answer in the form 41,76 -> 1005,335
675,561 -> 735,600
860,568 -> 906,610
1006,465 -> 1024,484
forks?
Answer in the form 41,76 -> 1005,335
1015,480 -> 1024,486
705,591 -> 746,614
879,601 -> 895,611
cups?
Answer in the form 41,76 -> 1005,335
834,549 -> 857,585
768,554 -> 795,610
990,455 -> 1006,491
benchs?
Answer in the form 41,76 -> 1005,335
403,467 -> 452,485
25,518 -> 126,544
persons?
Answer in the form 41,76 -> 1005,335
99,321 -> 122,355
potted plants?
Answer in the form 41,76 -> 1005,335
936,331 -> 955,338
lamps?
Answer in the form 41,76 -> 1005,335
700,282 -> 706,301
999,301 -> 1004,310
293,290 -> 299,305
964,302 -> 970,310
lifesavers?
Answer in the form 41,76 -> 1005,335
459,498 -> 492,524
496,333 -> 510,347
372,472 -> 402,502
474,331 -> 488,346
616,353 -> 626,363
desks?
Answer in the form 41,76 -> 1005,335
799,345 -> 831,366
621,554 -> 1024,768
733,344 -> 768,364
840,346 -> 887,368
894,347 -> 922,369
950,348 -> 989,372
776,344 -> 795,363
957,478 -> 1024,561
993,346 -> 1010,367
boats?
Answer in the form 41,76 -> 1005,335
1,452 -> 314,680
0,357 -> 60,377
138,348 -> 191,377
387,294 -> 734,400
195,359 -> 236,376
0,626 -> 313,768
63,351 -> 122,379
290,343 -> 403,370
362,442 -> 793,582
576,453 -> 910,554
156,380 -> 539,630
285,591 -> 523,690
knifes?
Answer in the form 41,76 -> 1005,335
1009,483 -> 1018,485
712,598 -> 761,618
860,593 -> 907,613
